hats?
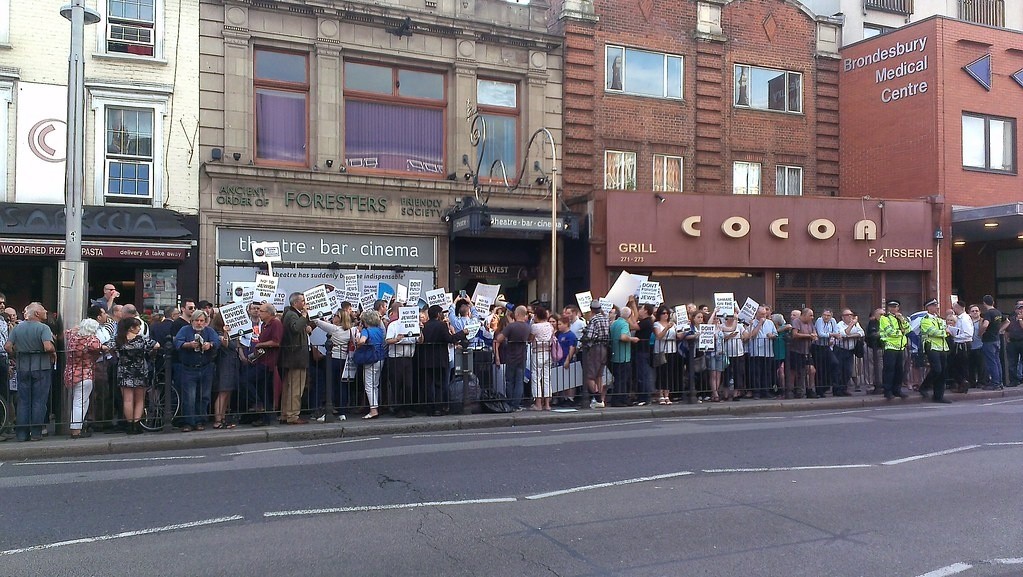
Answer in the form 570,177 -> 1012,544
957,300 -> 966,307
886,300 -> 900,307
1014,304 -> 1023,309
591,299 -> 601,309
924,298 -> 939,308
197,301 -> 213,309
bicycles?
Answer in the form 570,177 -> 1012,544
139,348 -> 181,432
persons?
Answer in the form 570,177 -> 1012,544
946,301 -> 974,392
879,300 -> 921,398
864,309 -> 885,388
1005,300 -> 1023,387
64,284 -> 792,438
979,295 -> 1011,390
919,299 -> 952,403
0,294 -> 58,440
791,308 -> 865,398
968,305 -> 984,387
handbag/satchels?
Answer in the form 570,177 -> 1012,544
722,355 -> 730,369
313,345 -> 325,361
854,341 -> 864,358
694,355 -> 707,373
349,328 -> 355,352
551,326 -> 563,362
353,327 -> 378,369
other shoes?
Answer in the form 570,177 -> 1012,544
0,378 -> 1023,443
652,353 -> 667,366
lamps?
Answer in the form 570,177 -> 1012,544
878,200 -> 887,209
535,177 -> 545,185
464,173 -> 471,181
654,193 -> 666,203
233,153 -> 242,162
326,159 -> 334,167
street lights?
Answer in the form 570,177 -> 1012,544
59,5 -> 101,262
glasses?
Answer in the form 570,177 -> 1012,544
0,301 -> 6,305
842,314 -> 854,317
185,307 -> 196,310
107,289 -> 116,291
